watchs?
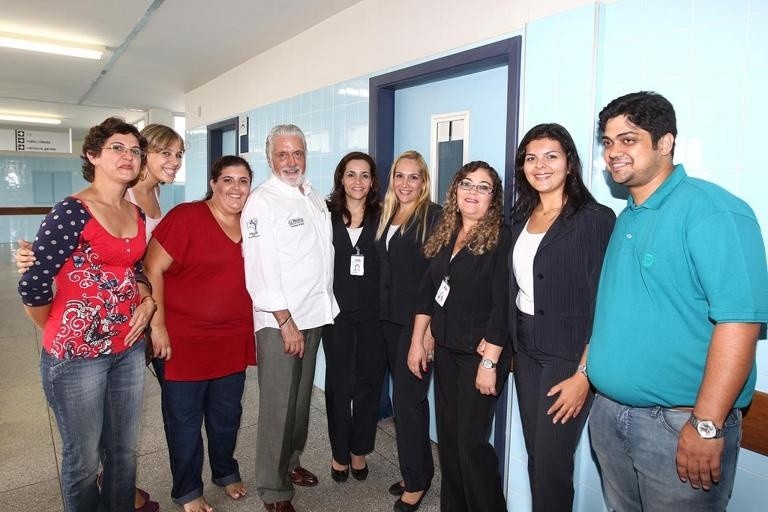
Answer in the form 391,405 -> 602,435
481,359 -> 496,369
687,413 -> 725,440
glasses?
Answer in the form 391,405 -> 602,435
101,144 -> 144,155
458,179 -> 493,195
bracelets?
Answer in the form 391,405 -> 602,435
576,365 -> 588,377
141,295 -> 158,310
279,314 -> 291,328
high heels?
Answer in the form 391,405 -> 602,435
388,480 -> 431,512
136,489 -> 160,511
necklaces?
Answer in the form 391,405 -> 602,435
536,200 -> 565,216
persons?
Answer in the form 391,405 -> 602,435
237,122 -> 343,511
319,150 -> 390,484
11,123 -> 186,512
14,114 -> 160,512
471,123 -> 617,512
405,160 -> 517,512
139,155 -> 259,512
584,87 -> 768,510
376,150 -> 456,510
355,264 -> 361,272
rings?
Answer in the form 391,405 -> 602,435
426,352 -> 433,359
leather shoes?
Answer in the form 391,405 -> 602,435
266,501 -> 295,512
331,461 -> 368,481
289,467 -> 317,487
